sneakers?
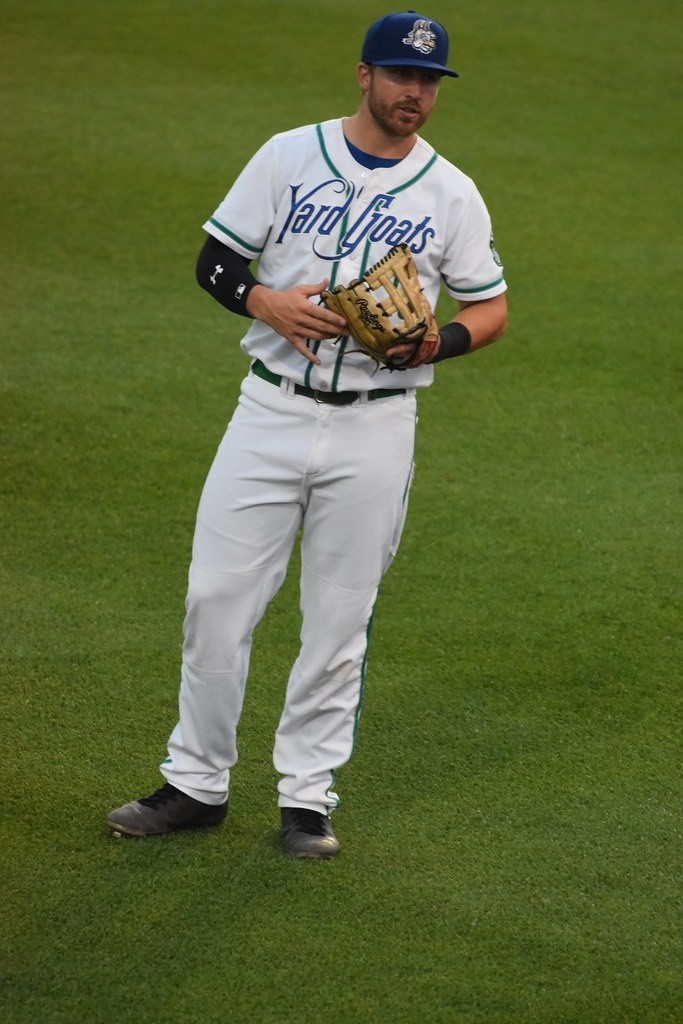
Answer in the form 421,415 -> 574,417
106,782 -> 228,838
280,807 -> 342,858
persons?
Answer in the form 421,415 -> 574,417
104,10 -> 505,857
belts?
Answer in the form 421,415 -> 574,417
251,359 -> 407,406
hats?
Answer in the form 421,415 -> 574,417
361,9 -> 460,78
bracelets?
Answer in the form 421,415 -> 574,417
425,322 -> 472,365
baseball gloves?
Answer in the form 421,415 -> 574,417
320,242 -> 443,371
